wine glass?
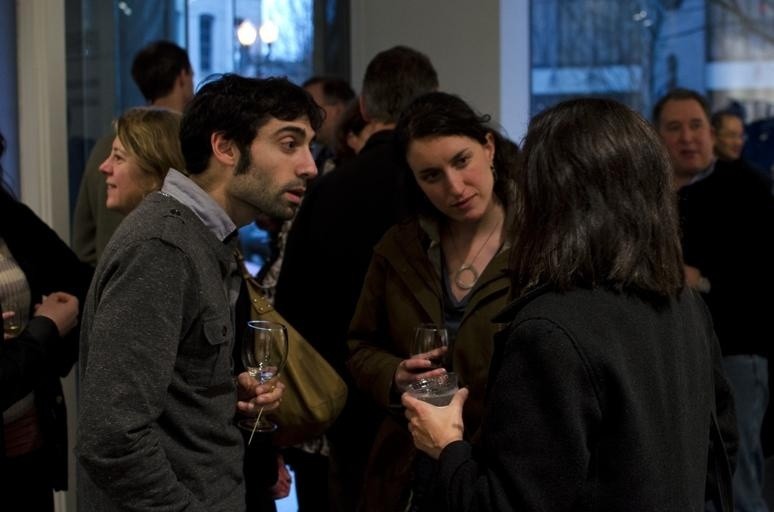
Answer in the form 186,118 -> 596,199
237,321 -> 289,433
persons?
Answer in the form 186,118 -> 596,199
73,74 -> 327,510
649,88 -> 772,510
401,93 -> 718,510
96,107 -> 186,212
71,39 -> 194,261
3,184 -> 95,511
264,47 -> 523,510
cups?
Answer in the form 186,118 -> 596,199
2,303 -> 23,333
411,326 -> 450,372
406,371 -> 459,409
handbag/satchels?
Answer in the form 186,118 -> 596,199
232,248 -> 349,438
